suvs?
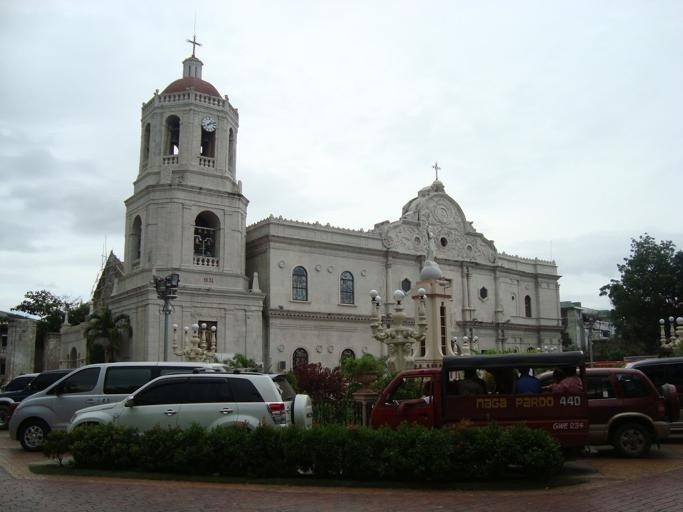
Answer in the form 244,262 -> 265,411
65,366 -> 314,472
529,367 -> 680,459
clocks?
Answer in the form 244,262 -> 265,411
202,117 -> 216,132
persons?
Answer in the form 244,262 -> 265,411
397,380 -> 433,412
449,367 -> 583,396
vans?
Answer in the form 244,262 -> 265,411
0,367 -> 76,431
6,360 -> 235,453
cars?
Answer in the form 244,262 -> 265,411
0,373 -> 40,392
586,357 -> 683,438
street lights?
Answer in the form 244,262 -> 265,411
582,310 -> 599,368
153,274 -> 180,362
171,322 -> 217,363
451,336 -> 479,356
369,288 -> 427,368
659,316 -> 683,351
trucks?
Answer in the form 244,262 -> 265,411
368,351 -> 589,457
